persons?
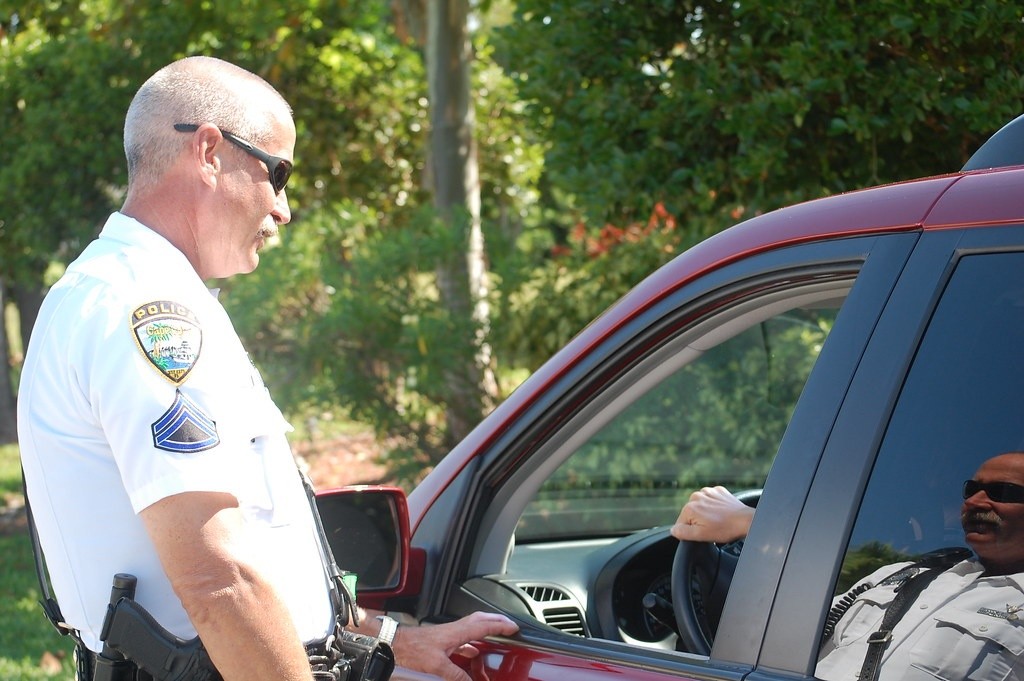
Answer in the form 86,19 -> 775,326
813,452 -> 1024,681
17,57 -> 520,681
670,486 -> 756,543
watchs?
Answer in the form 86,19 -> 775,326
376,615 -> 400,646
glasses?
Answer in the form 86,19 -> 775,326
962,480 -> 1024,503
174,122 -> 293,196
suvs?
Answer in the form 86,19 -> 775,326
309,113 -> 1023,680
